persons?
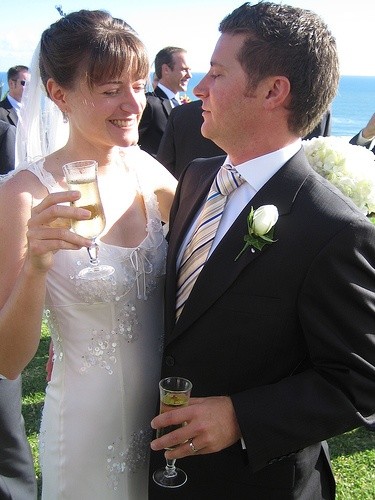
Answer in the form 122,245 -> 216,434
0,118 -> 41,500
0,66 -> 31,128
137,47 -> 192,160
350,112 -> 375,157
47,3 -> 375,499
0,8 -> 178,500
154,100 -> 228,179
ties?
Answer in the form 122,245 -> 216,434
174,164 -> 247,327
171,98 -> 179,107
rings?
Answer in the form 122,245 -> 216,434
189,438 -> 198,452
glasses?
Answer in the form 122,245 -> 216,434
13,79 -> 25,86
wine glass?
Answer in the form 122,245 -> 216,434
152,378 -> 193,489
62,160 -> 115,281
